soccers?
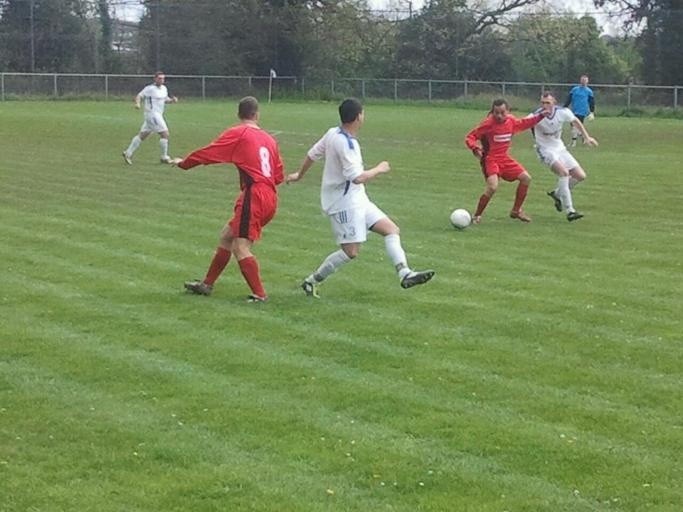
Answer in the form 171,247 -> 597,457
450,208 -> 471,229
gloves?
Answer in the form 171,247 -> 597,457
586,112 -> 595,122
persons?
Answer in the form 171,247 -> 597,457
562,75 -> 595,148
285,99 -> 435,299
122,71 -> 178,166
168,96 -> 284,304
464,99 -> 551,225
523,91 -> 598,221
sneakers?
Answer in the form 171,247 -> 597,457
546,190 -> 563,212
509,208 -> 532,223
244,294 -> 267,304
183,281 -> 214,297
470,216 -> 481,224
160,156 -> 174,164
300,278 -> 321,300
567,210 -> 584,222
399,268 -> 435,289
122,151 -> 132,166
